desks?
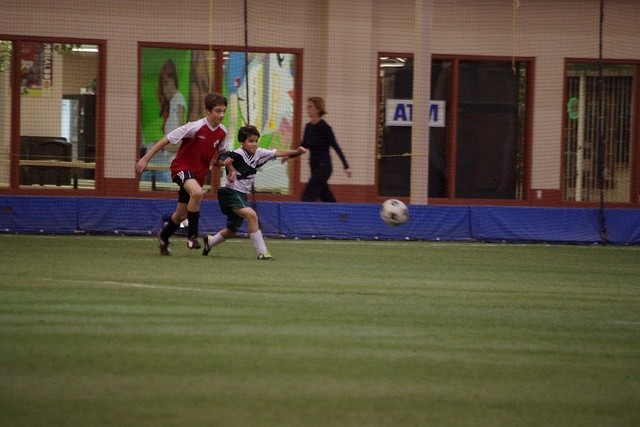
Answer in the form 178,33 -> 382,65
18,159 -> 95,188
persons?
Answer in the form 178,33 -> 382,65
282,95 -> 353,203
158,64 -> 188,160
134,91 -> 230,248
202,124 -> 307,260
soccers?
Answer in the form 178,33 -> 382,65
379,199 -> 409,226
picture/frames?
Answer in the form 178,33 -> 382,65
136,41 -> 303,203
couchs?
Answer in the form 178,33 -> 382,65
18,136 -> 72,185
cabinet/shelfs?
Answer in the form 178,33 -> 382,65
77,96 -> 95,161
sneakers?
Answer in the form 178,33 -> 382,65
187,239 -> 201,249
158,228 -> 170,256
203,235 -> 212,256
257,252 -> 274,260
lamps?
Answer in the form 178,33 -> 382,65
71,44 -> 98,52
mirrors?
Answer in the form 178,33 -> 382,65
0,35 -> 107,196
